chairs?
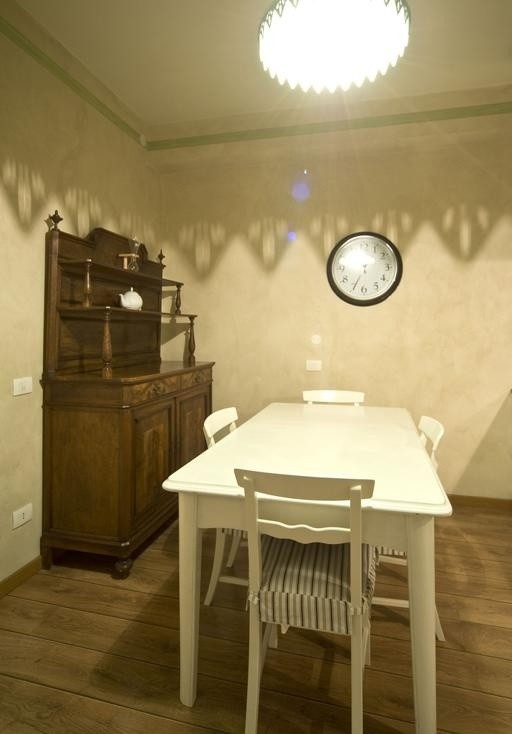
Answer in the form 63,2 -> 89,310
202,404 -> 248,606
372,414 -> 446,642
302,389 -> 364,407
233,467 -> 376,734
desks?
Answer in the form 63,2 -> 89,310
161,401 -> 453,733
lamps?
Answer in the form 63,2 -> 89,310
257,0 -> 411,93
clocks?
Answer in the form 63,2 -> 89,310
327,231 -> 403,305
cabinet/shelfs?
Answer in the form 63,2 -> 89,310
40,209 -> 215,578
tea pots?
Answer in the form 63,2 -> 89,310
117,286 -> 144,312
